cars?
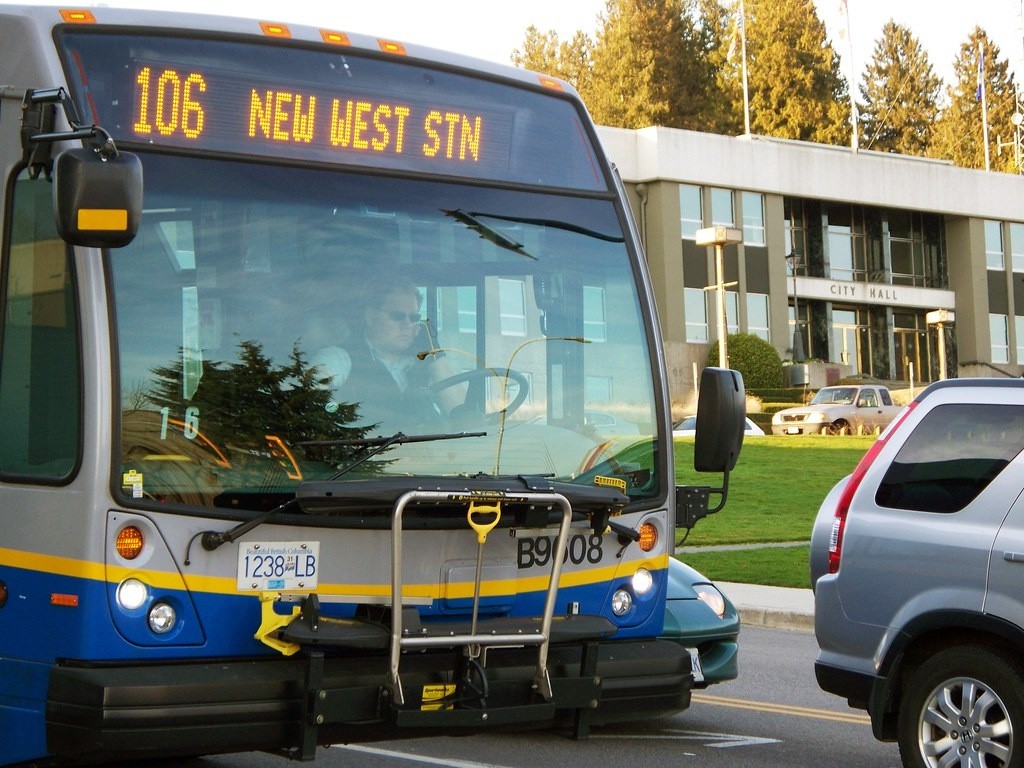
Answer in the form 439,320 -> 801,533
664,561 -> 743,695
671,415 -> 765,440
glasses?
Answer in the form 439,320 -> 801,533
376,306 -> 421,322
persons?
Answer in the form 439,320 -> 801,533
308,275 -> 470,436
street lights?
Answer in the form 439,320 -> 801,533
417,333 -> 594,476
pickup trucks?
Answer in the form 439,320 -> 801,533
770,379 -> 904,441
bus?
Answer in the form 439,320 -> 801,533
0,5 -> 750,768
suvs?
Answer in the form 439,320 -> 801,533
805,375 -> 1024,768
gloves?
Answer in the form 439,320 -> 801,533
416,320 -> 445,362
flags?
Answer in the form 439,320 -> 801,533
835,0 -> 850,41
724,6 -> 742,59
976,55 -> 985,99
